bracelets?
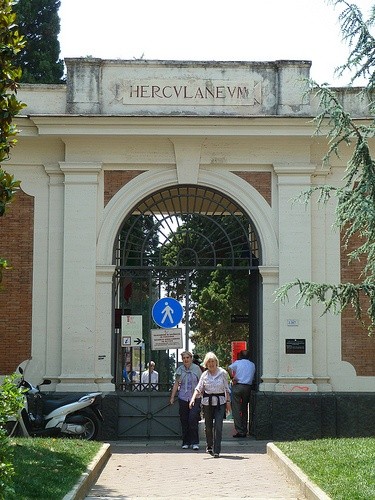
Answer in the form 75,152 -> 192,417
226,400 -> 230,402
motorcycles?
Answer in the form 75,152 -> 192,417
1,365 -> 106,441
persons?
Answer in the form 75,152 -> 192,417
170,351 -> 202,449
228,350 -> 255,438
141,361 -> 158,390
193,354 -> 204,372
123,363 -> 139,383
189,352 -> 231,457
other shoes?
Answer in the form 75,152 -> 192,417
193,444 -> 200,450
232,433 -> 246,439
182,445 -> 189,448
206,448 -> 212,453
214,453 -> 219,456
